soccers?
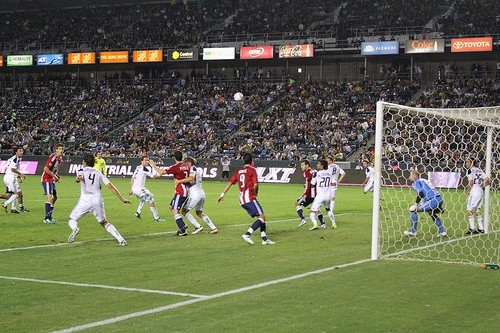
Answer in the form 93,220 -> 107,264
234,92 -> 243,101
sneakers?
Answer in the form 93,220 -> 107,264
319,224 -> 326,229
309,225 -> 318,231
68,228 -> 79,243
1,202 -> 8,213
298,219 -> 306,227
121,240 -> 127,246
11,209 -> 20,214
154,217 -> 165,222
465,229 -> 476,234
474,229 -> 484,234
403,230 -> 418,236
261,239 -> 275,244
435,231 -> 447,237
173,229 -> 188,236
20,206 -> 30,212
51,219 -> 58,224
192,225 -> 203,235
43,218 -> 52,224
135,212 -> 142,219
177,224 -> 189,232
328,224 -> 337,229
209,227 -> 219,234
241,234 -> 255,244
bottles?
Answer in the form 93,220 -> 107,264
482,264 -> 499,270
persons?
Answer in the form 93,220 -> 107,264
41,143 -> 65,224
129,156 -> 165,222
466,157 -> 485,234
147,151 -> 218,236
362,160 -> 383,196
68,155 -> 133,245
404,171 -> 447,236
327,157 -> 346,189
217,153 -> 275,244
0,0 -> 500,52
0,147 -> 30,213
296,160 -> 326,228
309,160 -> 337,231
93,152 -> 107,189
0,58 -> 500,182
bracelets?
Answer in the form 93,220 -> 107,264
221,193 -> 225,197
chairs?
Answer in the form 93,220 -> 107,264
107,127 -> 124,143
